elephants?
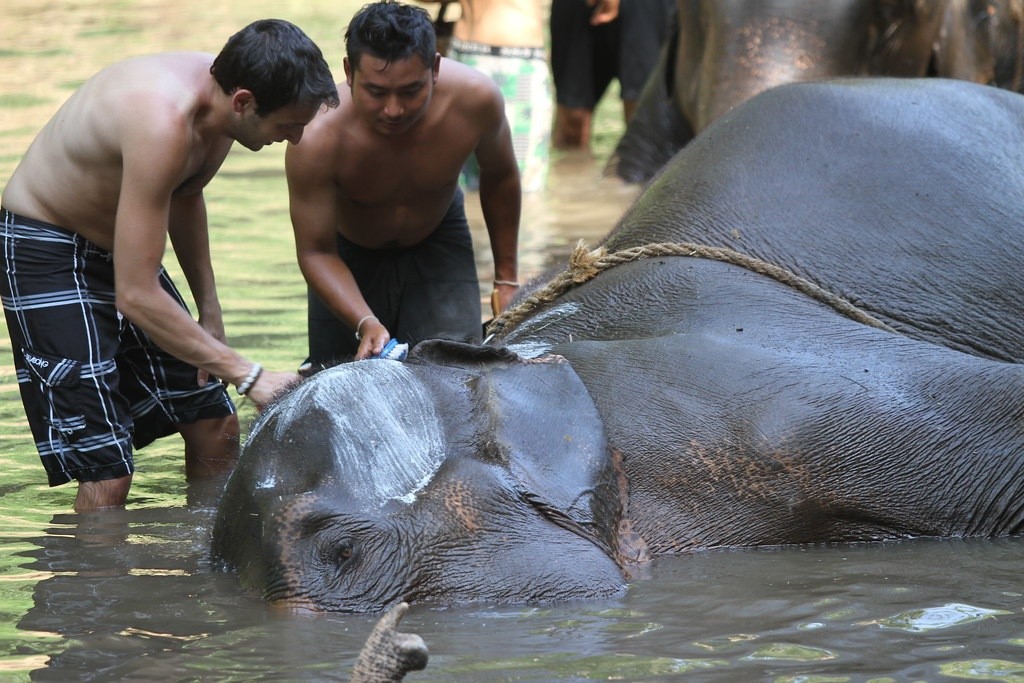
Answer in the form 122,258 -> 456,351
547,0 -> 1024,183
209,80 -> 1023,682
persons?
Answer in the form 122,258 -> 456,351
442,0 -> 619,192
0,18 -> 339,510
285,2 -> 523,363
550,0 -> 673,148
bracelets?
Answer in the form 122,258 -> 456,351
355,316 -> 379,340
492,278 -> 519,288
236,362 -> 263,395
